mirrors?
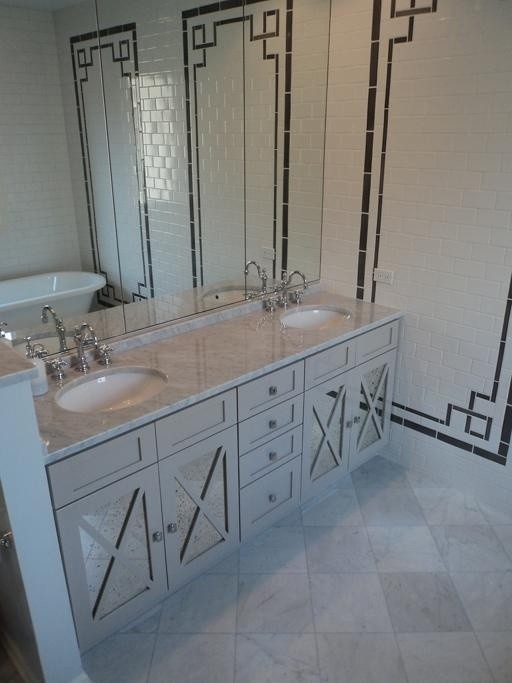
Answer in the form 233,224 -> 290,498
0,0 -> 331,359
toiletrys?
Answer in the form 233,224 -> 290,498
26,347 -> 49,396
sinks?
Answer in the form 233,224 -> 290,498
54,365 -> 169,414
201,285 -> 261,305
13,331 -> 76,357
280,305 -> 353,330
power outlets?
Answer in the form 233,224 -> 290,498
374,269 -> 393,284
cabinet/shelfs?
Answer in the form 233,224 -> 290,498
47,318 -> 400,656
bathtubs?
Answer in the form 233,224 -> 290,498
0,271 -> 107,327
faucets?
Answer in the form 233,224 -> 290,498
281,270 -> 307,306
243,261 -> 268,294
74,323 -> 98,374
40,305 -> 70,354
